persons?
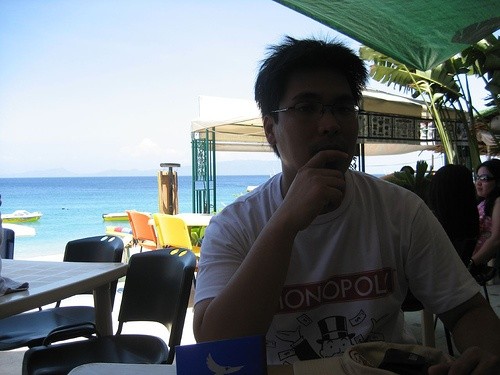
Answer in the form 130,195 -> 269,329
192,35 -> 500,375
394,159 -> 500,272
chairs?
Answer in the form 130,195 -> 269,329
0,227 -> 15,259
125,209 -> 158,253
0,235 -> 124,351
22,247 -> 196,375
434,243 -> 500,336
151,213 -> 201,291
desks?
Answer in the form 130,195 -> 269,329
0,258 -> 130,338
149,212 -> 214,242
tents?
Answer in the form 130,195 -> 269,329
192,89 -> 473,214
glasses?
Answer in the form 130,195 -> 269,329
271,99 -> 365,121
474,174 -> 495,183
